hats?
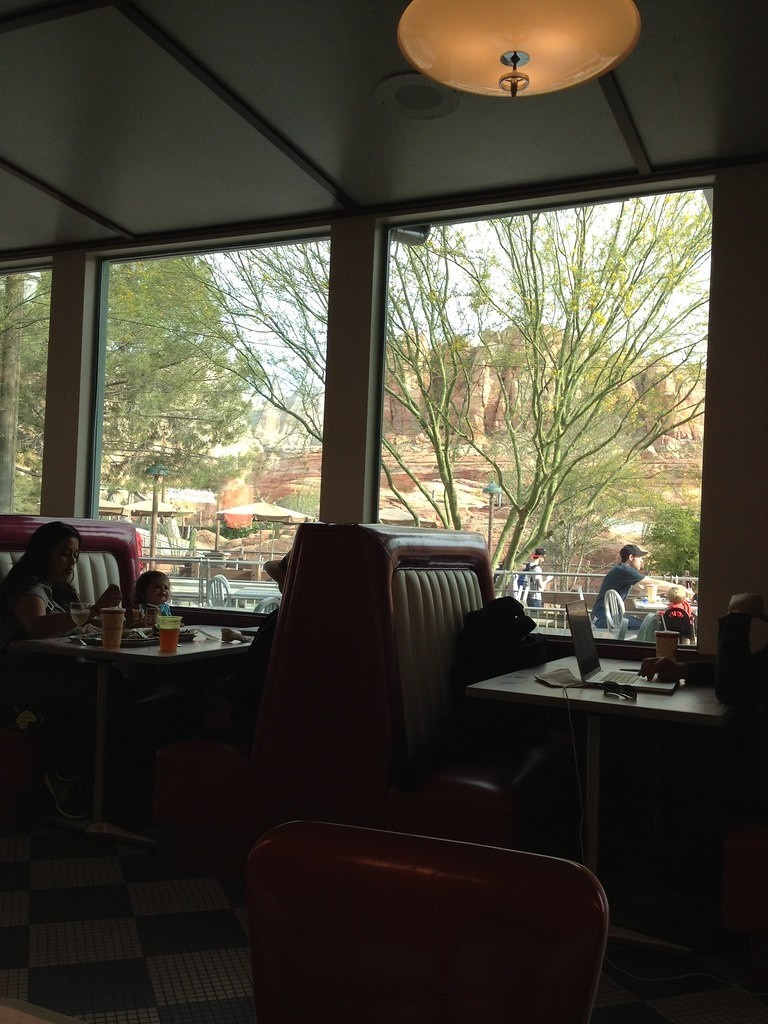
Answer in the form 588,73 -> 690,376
535,548 -> 546,555
263,549 -> 291,583
620,545 -> 648,562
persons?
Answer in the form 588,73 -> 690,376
133,570 -> 185,628
0,521 -> 122,819
517,548 -> 554,611
590,544 -> 695,630
603,592 -> 768,889
658,587 -> 693,625
493,562 -> 503,584
169,515 -> 180,538
202,548 -> 292,729
449,597 -> 572,855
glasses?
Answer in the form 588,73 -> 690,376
599,681 -> 637,700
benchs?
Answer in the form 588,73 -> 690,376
0,514 -> 768,1001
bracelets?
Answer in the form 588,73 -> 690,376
93,604 -> 99,616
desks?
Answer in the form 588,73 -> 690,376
630,598 -> 698,622
467,656 -> 733,956
233,586 -> 282,609
11,624 -> 260,847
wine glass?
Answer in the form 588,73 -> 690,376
69,602 -> 91,646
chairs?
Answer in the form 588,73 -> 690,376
577,584 -> 695,646
205,573 -> 282,614
238,817 -> 609,1024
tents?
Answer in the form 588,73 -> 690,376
378,507 -> 439,528
216,502 -> 317,524
99,499 -> 124,516
122,500 -> 194,516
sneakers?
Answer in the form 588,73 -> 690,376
46,771 -> 88,818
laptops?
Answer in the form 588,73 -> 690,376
566,600 -> 679,694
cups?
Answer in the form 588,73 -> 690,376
100,608 -> 126,650
653,629 -> 681,664
646,585 -> 658,603
157,615 -> 183,653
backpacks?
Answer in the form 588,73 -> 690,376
517,563 -> 539,590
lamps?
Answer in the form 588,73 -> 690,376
391,224 -> 431,245
398,0 -> 642,98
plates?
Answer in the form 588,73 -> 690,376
179,632 -> 198,642
68,628 -> 159,648
